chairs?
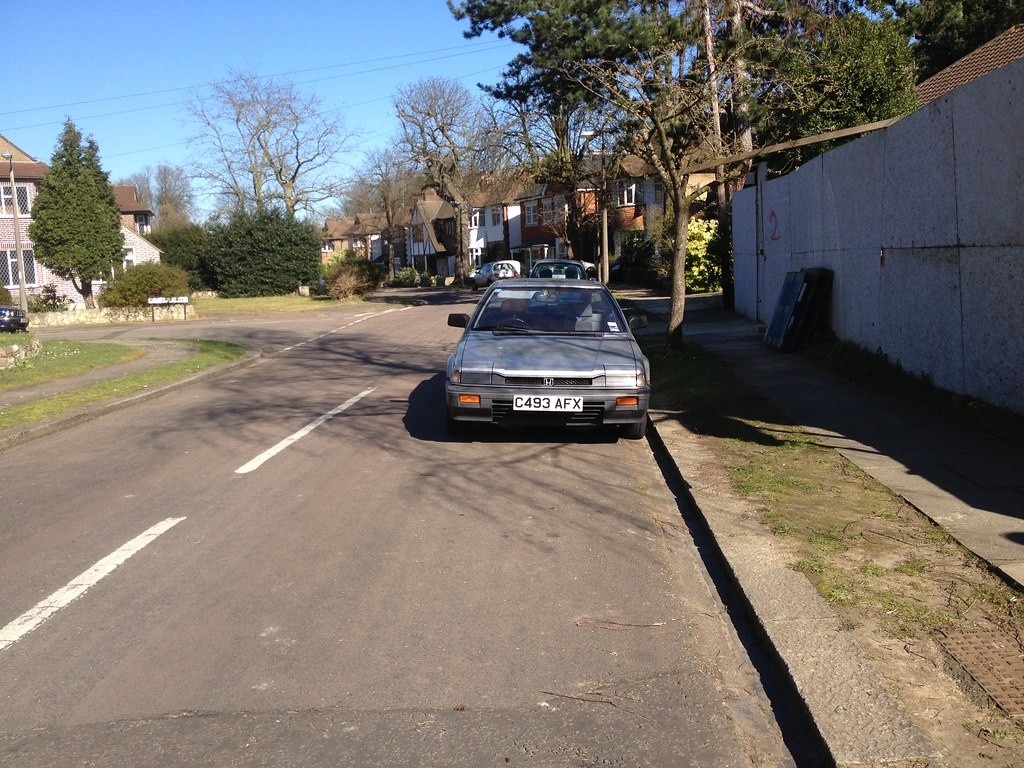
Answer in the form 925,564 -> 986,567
562,302 -> 603,331
501,300 -> 527,318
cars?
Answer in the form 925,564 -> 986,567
472,262 -> 519,289
445,279 -> 650,440
527,260 -> 588,280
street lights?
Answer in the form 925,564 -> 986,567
579,129 -> 610,285
1,151 -> 29,319
